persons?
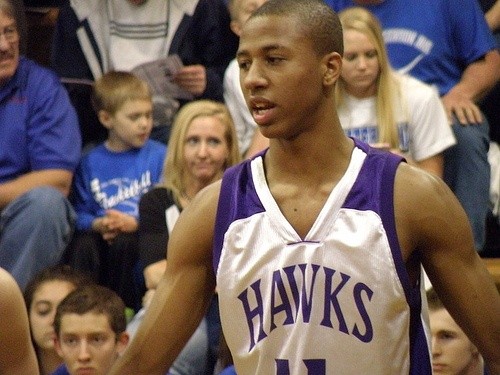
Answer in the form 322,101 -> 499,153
331,5 -> 456,179
106,0 -> 500,375
26,265 -> 130,375
324,0 -> 499,254
53,0 -> 239,150
224,0 -> 268,159
426,286 -> 499,375
0,0 -> 82,290
138,99 -> 241,375
70,72 -> 168,279
